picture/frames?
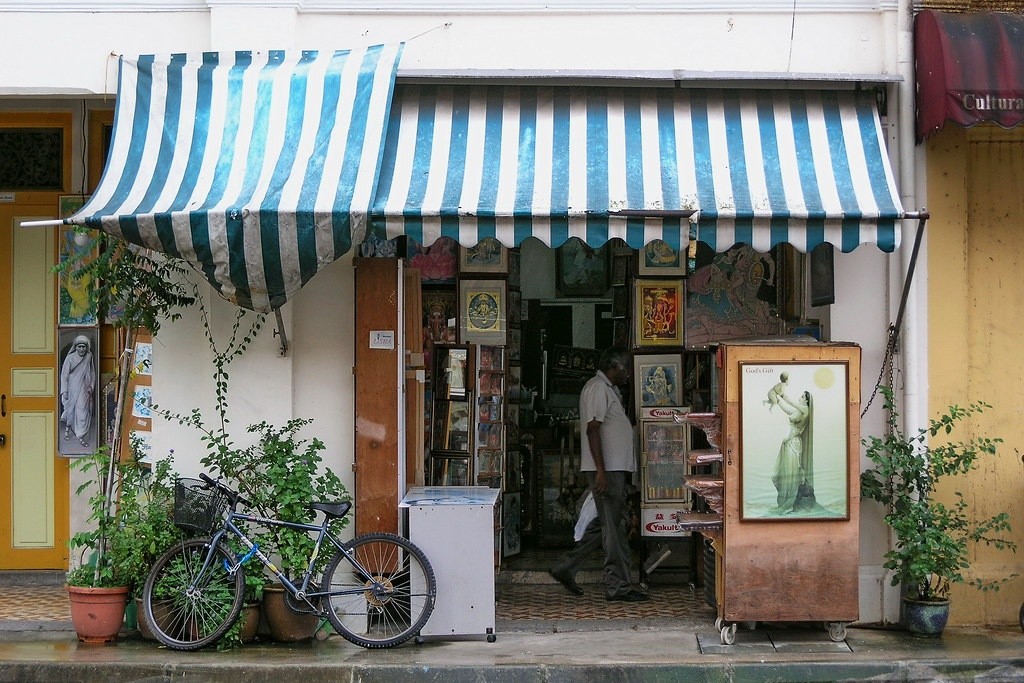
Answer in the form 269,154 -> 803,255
635,279 -> 683,347
555,237 -> 628,345
634,354 -> 683,420
738,360 -> 851,523
637,240 -> 688,275
55,325 -> 100,459
57,196 -> 100,327
430,238 -> 523,561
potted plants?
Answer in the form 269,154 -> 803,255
47,198 -> 355,652
859,385 -> 1017,638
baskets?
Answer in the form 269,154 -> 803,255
174,476 -> 234,532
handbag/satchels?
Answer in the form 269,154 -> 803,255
88,386 -> 96,417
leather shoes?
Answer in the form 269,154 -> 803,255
605,588 -> 651,603
547,564 -> 584,596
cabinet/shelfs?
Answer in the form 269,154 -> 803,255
398,486 -> 502,642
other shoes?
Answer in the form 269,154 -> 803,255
80,439 -> 89,448
64,431 -> 70,441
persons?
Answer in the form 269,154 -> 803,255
549,347 -> 649,603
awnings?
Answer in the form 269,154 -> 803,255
66,39 -> 404,311
357,87 -> 904,253
915,8 -> 1024,143
